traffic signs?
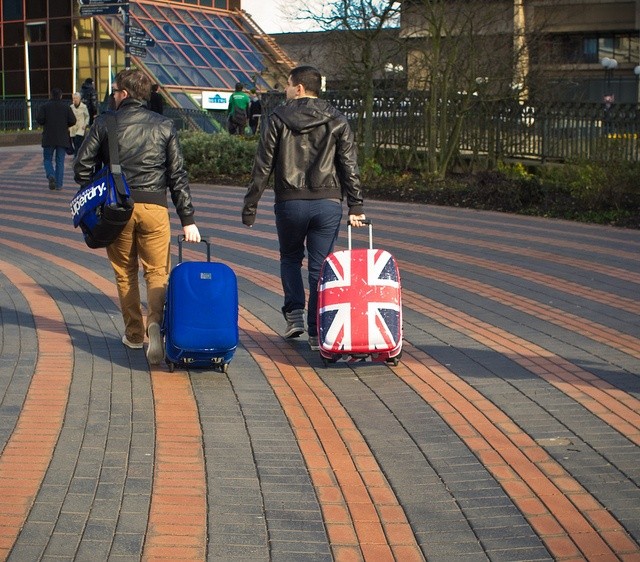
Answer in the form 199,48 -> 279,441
79,4 -> 127,16
129,37 -> 155,46
129,47 -> 147,56
129,26 -> 146,35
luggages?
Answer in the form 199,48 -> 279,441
316,218 -> 403,366
160,234 -> 239,373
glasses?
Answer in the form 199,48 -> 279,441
111,87 -> 125,95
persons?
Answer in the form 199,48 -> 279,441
267,83 -> 281,98
68,92 -> 91,159
249,89 -> 259,107
79,78 -> 98,127
72,68 -> 202,366
250,98 -> 264,134
36,87 -> 77,191
240,65 -> 368,352
146,83 -> 163,114
226,83 -> 251,136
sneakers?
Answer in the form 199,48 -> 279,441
146,322 -> 165,365
284,308 -> 304,338
308,336 -> 319,350
121,335 -> 144,349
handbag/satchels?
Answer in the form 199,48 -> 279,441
70,111 -> 134,248
231,95 -> 247,127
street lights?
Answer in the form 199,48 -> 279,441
635,64 -> 639,131
384,62 -> 404,102
601,57 -> 617,136
